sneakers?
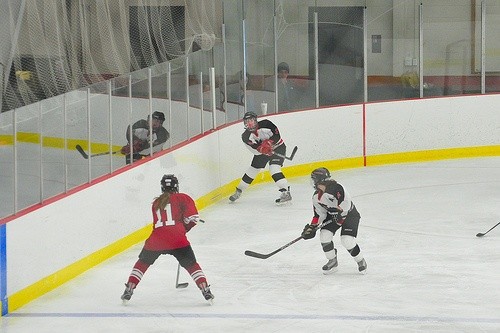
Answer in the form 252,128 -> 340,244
228,187 -> 242,202
274,186 -> 293,205
356,259 -> 368,274
198,282 -> 215,300
322,249 -> 339,275
121,283 -> 135,301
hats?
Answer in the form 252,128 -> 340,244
278,62 -> 289,73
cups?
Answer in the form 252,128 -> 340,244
260,102 -> 267,115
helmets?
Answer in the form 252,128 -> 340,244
310,167 -> 331,184
160,174 -> 179,192
148,111 -> 165,131
243,112 -> 258,131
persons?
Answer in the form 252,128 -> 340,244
301,167 -> 368,275
277,62 -> 298,110
229,112 -> 292,206
120,174 -> 215,305
121,111 -> 170,166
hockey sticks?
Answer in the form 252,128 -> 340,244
176,262 -> 189,289
75,144 -> 135,160
476,220 -> 500,237
257,143 -> 298,161
244,215 -> 341,260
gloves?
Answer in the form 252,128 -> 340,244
301,224 -> 317,240
258,140 -> 273,154
331,215 -> 342,224
120,146 -> 137,157
